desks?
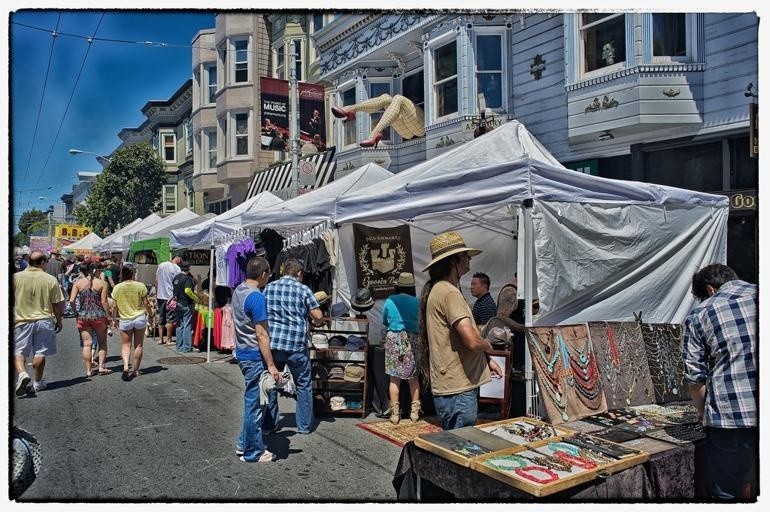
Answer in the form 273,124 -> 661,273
392,399 -> 705,502
193,303 -> 215,353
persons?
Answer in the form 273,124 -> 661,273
308,110 -> 321,137
260,257 -> 323,434
13,249 -> 230,397
470,272 -> 497,330
231,256 -> 283,463
496,271 -> 525,378
330,93 -> 426,149
420,230 -> 504,431
382,271 -> 421,424
680,263 -> 757,501
270,131 -> 287,150
265,118 -> 277,131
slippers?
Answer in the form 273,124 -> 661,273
99,368 -> 114,376
87,369 -> 95,377
240,450 -> 277,462
236,444 -> 268,454
91,360 -> 99,367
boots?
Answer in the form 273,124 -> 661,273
410,400 -> 422,423
390,402 -> 399,424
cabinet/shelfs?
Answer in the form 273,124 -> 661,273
307,313 -> 370,419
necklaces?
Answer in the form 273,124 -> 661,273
455,322 -> 690,484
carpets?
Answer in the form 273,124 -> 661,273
358,417 -> 496,448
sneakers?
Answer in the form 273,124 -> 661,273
185,347 -> 200,353
15,373 -> 31,395
262,424 -> 278,434
32,377 -> 47,392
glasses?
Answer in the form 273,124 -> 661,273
265,271 -> 272,277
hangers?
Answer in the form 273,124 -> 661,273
218,227 -> 252,246
225,297 -> 232,308
280,219 -> 333,252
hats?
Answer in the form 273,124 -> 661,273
181,262 -> 191,268
51,250 -> 61,256
394,272 -> 416,286
176,252 -> 187,261
422,230 -> 482,273
304,288 -> 375,412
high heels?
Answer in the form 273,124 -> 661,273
360,134 -> 382,150
331,107 -> 356,122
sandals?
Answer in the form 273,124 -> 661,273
134,369 -> 144,377
122,368 -> 130,380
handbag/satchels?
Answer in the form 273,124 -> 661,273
166,295 -> 177,311
